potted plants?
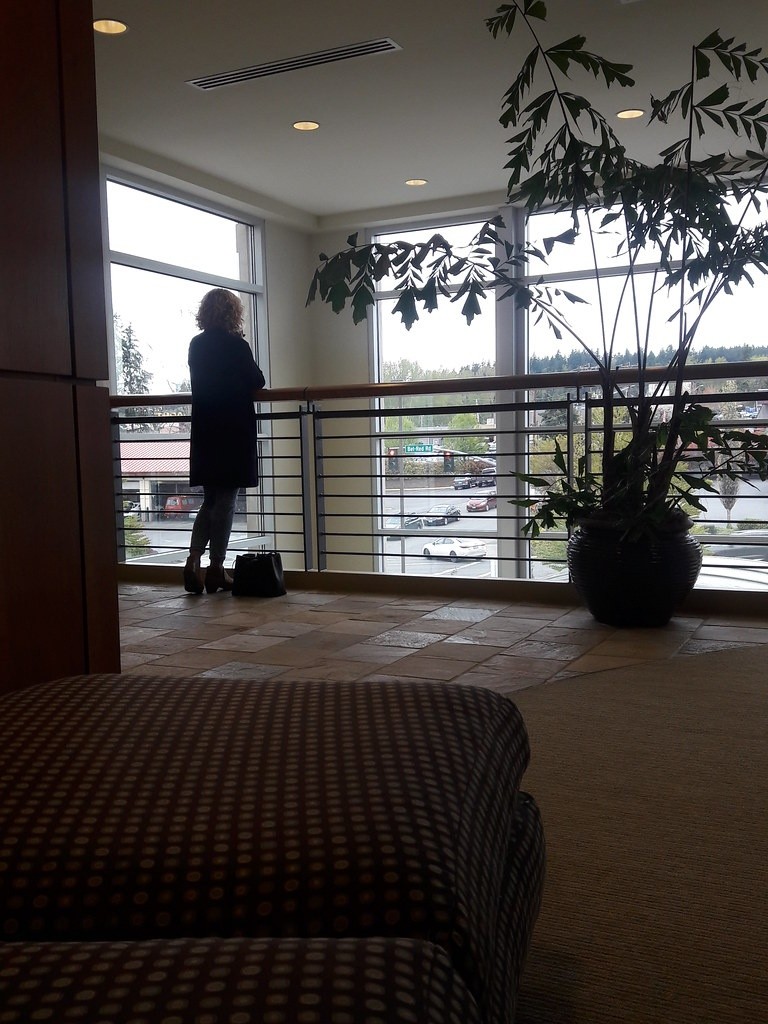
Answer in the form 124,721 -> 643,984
304,0 -> 767,628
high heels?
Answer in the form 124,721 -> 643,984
206,565 -> 236,593
182,558 -> 203,595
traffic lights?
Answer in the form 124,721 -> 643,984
444,451 -> 455,473
387,448 -> 398,471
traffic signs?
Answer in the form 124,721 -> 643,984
405,444 -> 432,454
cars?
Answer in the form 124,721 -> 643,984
122,494 -> 205,518
423,505 -> 461,526
384,513 -> 423,541
454,473 -> 476,490
467,490 -> 497,512
422,537 -> 486,563
476,475 -> 496,487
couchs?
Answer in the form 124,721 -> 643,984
1,676 -> 548,1023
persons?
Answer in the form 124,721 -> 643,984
180,286 -> 269,602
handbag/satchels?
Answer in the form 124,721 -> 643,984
236,549 -> 288,596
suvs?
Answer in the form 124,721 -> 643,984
482,467 -> 496,476
